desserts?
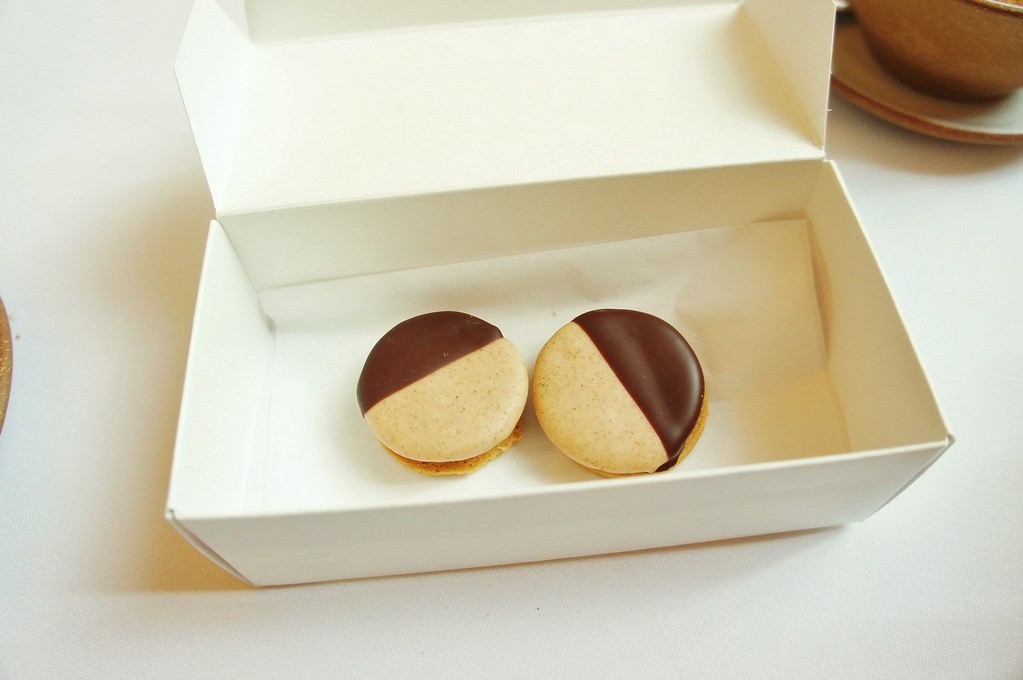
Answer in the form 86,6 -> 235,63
532,308 -> 708,478
356,310 -> 529,474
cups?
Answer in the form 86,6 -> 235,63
845,0 -> 1023,103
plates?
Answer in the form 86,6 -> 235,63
830,12 -> 1023,146
